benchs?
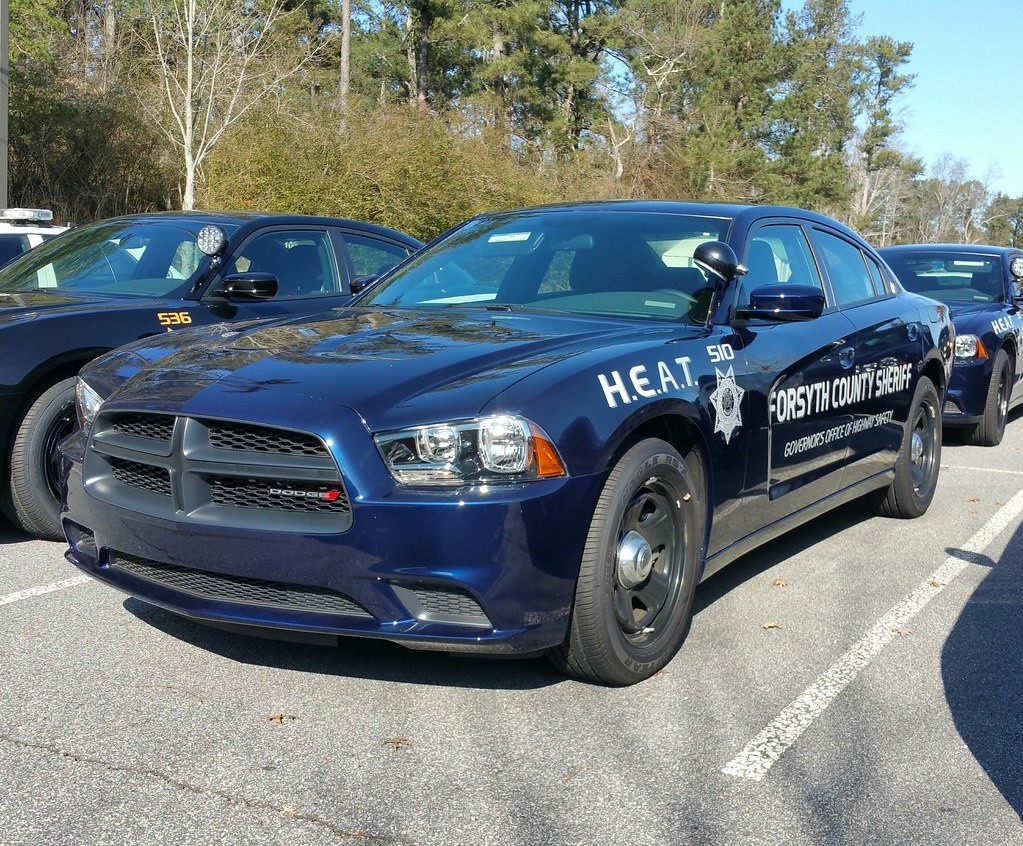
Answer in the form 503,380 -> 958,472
913,276 -> 971,290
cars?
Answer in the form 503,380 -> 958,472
61,201 -> 957,687
1,209 -> 478,542
875,243 -> 1023,446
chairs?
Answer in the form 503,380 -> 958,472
275,245 -> 324,296
741,238 -> 780,308
567,232 -> 675,294
895,271 -> 919,292
971,272 -> 1001,296
